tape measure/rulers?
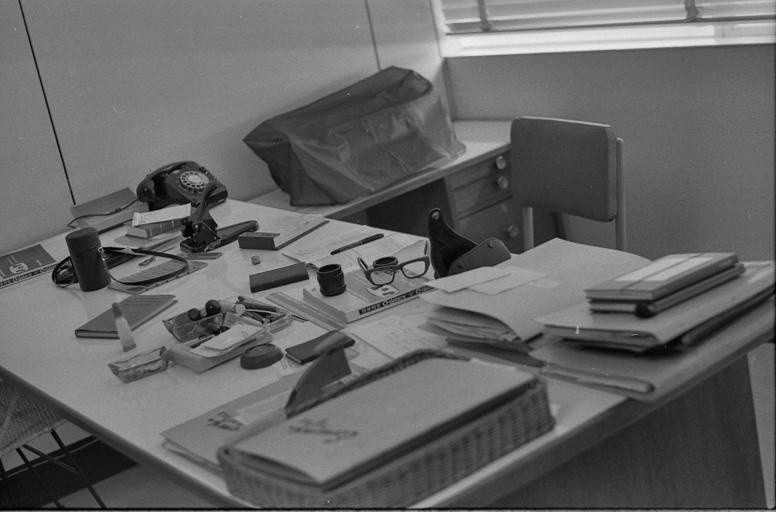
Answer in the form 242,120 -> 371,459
266,291 -> 345,332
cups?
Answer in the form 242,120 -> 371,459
65,227 -> 110,292
317,264 -> 347,296
373,257 -> 398,268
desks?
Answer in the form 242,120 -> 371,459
243,119 -> 568,254
0,198 -> 775,509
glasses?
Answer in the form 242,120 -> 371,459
356,240 -> 432,287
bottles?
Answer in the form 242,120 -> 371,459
112,302 -> 137,352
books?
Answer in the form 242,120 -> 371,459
225,356 -> 536,492
301,262 -> 434,324
583,250 -> 745,315
0,243 -> 59,289
70,186 -> 150,233
74,292 -> 178,341
123,218 -> 181,240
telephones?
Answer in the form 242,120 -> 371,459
137,161 -> 228,211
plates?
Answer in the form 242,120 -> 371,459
241,342 -> 284,370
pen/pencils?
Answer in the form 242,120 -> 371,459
331,234 -> 384,255
187,296 -> 287,337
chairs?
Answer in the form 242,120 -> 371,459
511,116 -> 626,253
